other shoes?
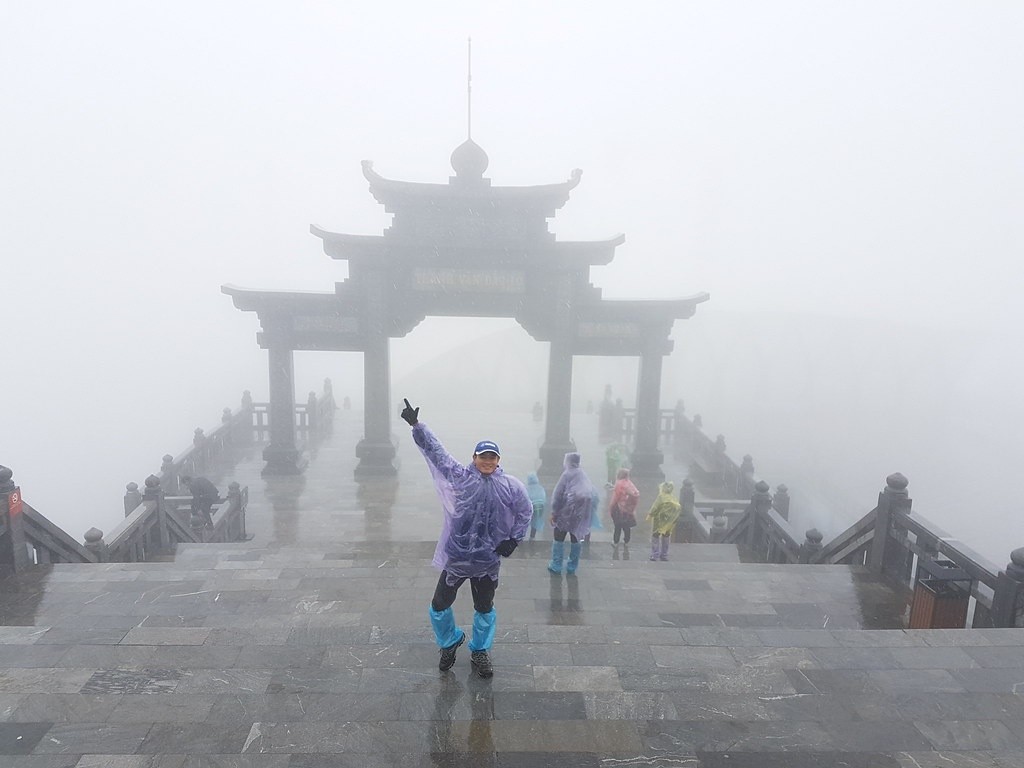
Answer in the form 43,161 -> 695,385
623,541 -> 629,546
611,541 -> 617,547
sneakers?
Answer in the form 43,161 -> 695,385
471,650 -> 493,677
439,633 -> 465,670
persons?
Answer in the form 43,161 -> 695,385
400,397 -> 532,678
582,485 -> 603,542
547,451 -> 594,574
606,467 -> 642,549
181,475 -> 220,529
519,471 -> 548,541
646,480 -> 682,562
603,442 -> 621,491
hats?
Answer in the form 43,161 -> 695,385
475,441 -> 500,458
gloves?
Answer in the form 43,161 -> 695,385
493,538 -> 517,556
400,398 -> 419,424
